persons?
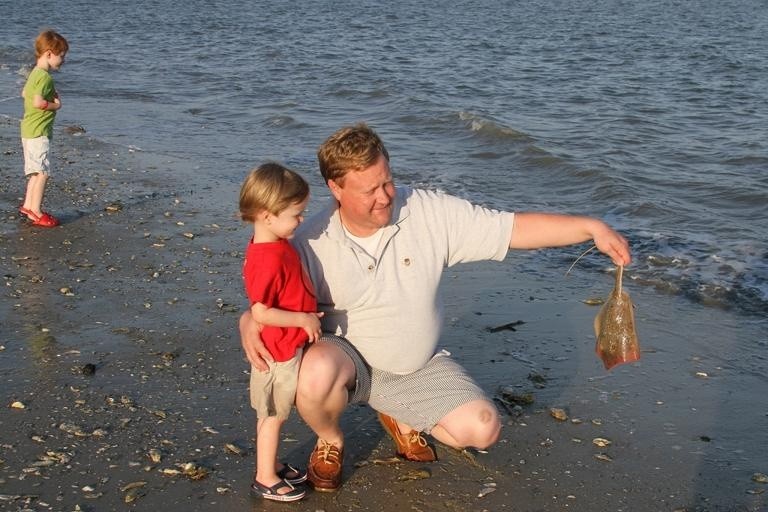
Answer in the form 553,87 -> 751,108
237,121 -> 632,495
18,29 -> 70,229
239,161 -> 324,502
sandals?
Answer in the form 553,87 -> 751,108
18,207 -> 58,226
250,461 -> 309,502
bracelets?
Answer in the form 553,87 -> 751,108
42,101 -> 48,110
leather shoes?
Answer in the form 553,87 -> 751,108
376,410 -> 437,462
307,440 -> 345,491
